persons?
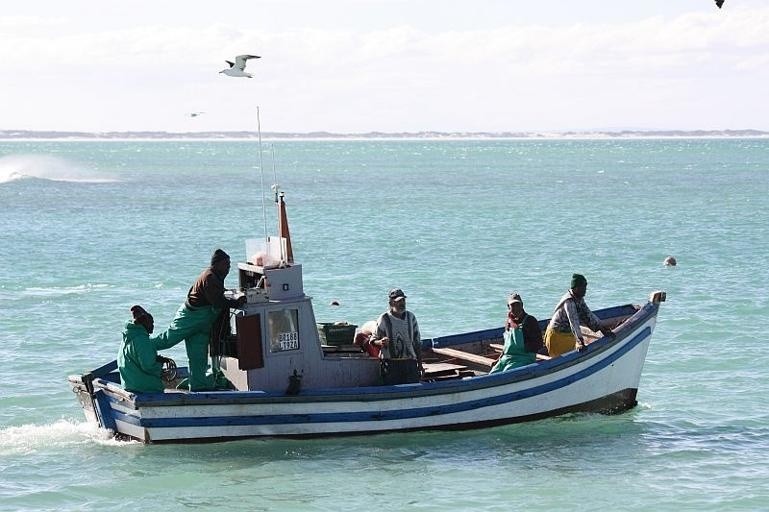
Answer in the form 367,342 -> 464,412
150,248 -> 248,391
542,273 -> 612,357
368,287 -> 423,386
118,306 -> 168,393
487,293 -> 542,377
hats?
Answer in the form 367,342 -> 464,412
389,288 -> 407,302
131,304 -> 149,324
211,249 -> 229,266
508,293 -> 522,305
570,274 -> 587,289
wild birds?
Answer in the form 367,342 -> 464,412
218,53 -> 261,79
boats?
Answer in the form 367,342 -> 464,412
67,104 -> 666,446
